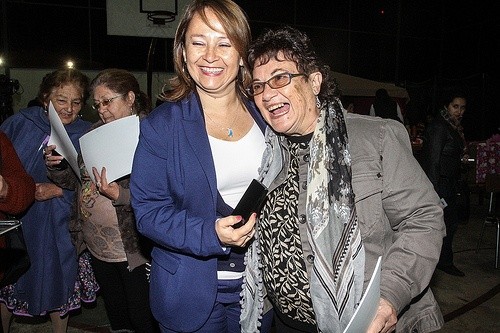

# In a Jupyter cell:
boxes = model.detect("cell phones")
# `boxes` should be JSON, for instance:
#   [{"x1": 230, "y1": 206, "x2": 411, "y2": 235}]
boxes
[{"x1": 229, "y1": 179, "x2": 268, "y2": 229}]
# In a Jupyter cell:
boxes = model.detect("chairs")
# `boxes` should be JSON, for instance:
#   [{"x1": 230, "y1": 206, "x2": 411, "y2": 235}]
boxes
[{"x1": 473, "y1": 174, "x2": 500, "y2": 270}]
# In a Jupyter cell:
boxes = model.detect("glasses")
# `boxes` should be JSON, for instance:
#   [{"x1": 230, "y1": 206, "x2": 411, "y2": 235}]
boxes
[
  {"x1": 246, "y1": 72, "x2": 305, "y2": 97},
  {"x1": 91, "y1": 93, "x2": 124, "y2": 109}
]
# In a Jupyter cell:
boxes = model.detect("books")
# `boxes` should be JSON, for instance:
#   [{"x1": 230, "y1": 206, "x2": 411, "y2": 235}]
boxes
[
  {"x1": 343, "y1": 255, "x2": 383, "y2": 333},
  {"x1": 46, "y1": 100, "x2": 139, "y2": 189}
]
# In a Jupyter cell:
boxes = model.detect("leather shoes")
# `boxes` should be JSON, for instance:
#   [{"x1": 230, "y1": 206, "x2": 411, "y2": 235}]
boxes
[{"x1": 440, "y1": 264, "x2": 464, "y2": 277}]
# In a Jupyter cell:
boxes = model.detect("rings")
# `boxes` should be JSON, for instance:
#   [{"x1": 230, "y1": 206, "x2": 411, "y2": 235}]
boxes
[
  {"x1": 43, "y1": 149, "x2": 51, "y2": 157},
  {"x1": 96, "y1": 182, "x2": 102, "y2": 188}
]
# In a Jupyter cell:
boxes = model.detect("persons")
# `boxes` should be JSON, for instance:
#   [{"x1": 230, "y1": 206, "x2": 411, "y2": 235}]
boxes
[
  {"x1": 0, "y1": 61, "x2": 176, "y2": 333},
  {"x1": 129, "y1": 0, "x2": 275, "y2": 333},
  {"x1": 238, "y1": 26, "x2": 444, "y2": 333},
  {"x1": 340, "y1": 88, "x2": 472, "y2": 277}
]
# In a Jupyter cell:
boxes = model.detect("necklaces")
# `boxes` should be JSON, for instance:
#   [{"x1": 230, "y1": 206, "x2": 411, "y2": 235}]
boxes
[{"x1": 202, "y1": 102, "x2": 245, "y2": 138}]
